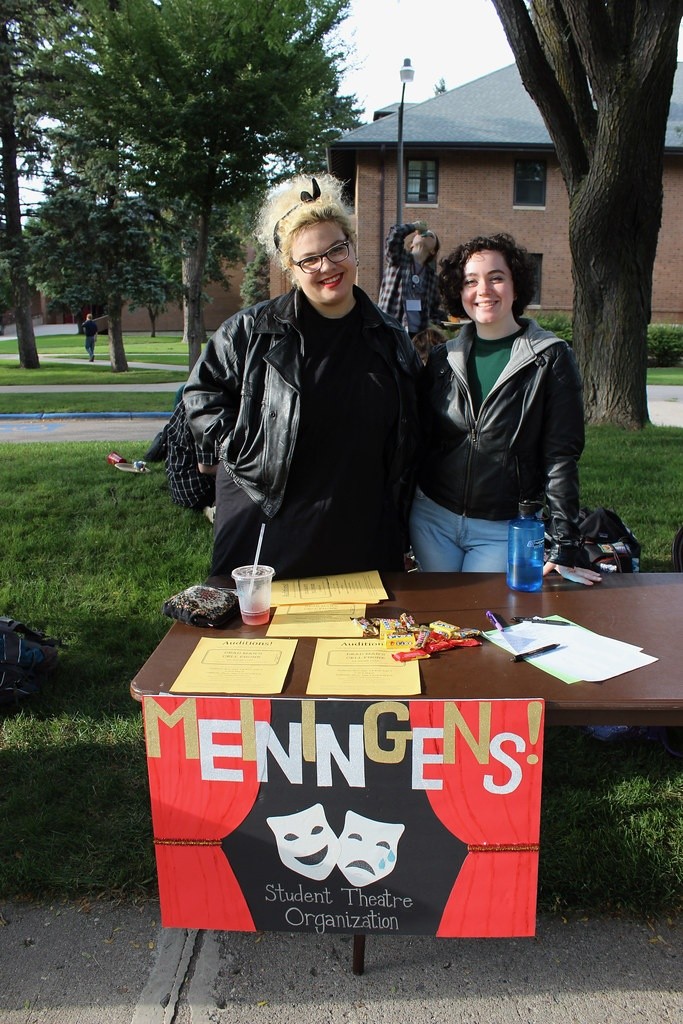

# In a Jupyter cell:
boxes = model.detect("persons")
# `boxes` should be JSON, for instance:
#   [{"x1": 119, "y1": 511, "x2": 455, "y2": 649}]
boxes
[
  {"x1": 182, "y1": 173, "x2": 426, "y2": 578},
  {"x1": 82, "y1": 314, "x2": 98, "y2": 362},
  {"x1": 409, "y1": 233, "x2": 602, "y2": 586},
  {"x1": 165, "y1": 221, "x2": 448, "y2": 511}
]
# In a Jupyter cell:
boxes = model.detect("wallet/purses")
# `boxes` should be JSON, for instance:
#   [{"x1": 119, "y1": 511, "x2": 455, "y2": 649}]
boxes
[{"x1": 165, "y1": 585, "x2": 237, "y2": 628}]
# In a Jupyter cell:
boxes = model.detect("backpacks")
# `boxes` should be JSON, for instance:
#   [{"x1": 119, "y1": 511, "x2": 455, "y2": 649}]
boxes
[
  {"x1": 0, "y1": 616, "x2": 58, "y2": 718},
  {"x1": 577, "y1": 507, "x2": 639, "y2": 574}
]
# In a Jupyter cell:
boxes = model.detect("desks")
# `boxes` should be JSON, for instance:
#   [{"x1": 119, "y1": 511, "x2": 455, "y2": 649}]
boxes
[{"x1": 129, "y1": 573, "x2": 683, "y2": 978}]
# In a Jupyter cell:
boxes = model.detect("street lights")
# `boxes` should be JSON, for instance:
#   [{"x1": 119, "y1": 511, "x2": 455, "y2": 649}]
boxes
[{"x1": 395, "y1": 57, "x2": 415, "y2": 232}]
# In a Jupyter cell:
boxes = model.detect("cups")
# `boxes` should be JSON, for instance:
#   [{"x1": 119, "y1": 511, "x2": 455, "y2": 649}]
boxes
[
  {"x1": 106, "y1": 451, "x2": 127, "y2": 464},
  {"x1": 232, "y1": 565, "x2": 276, "y2": 625}
]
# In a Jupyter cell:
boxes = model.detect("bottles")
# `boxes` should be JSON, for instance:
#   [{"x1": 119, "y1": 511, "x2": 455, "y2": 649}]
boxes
[{"x1": 506, "y1": 500, "x2": 552, "y2": 592}]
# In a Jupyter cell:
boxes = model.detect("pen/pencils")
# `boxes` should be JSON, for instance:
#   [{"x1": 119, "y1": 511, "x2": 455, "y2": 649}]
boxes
[
  {"x1": 509, "y1": 644, "x2": 561, "y2": 663},
  {"x1": 485, "y1": 611, "x2": 505, "y2": 633},
  {"x1": 532, "y1": 620, "x2": 570, "y2": 626}
]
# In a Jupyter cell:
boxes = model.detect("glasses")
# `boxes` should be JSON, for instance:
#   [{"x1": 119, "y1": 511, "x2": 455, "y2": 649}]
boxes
[{"x1": 290, "y1": 234, "x2": 350, "y2": 274}]
